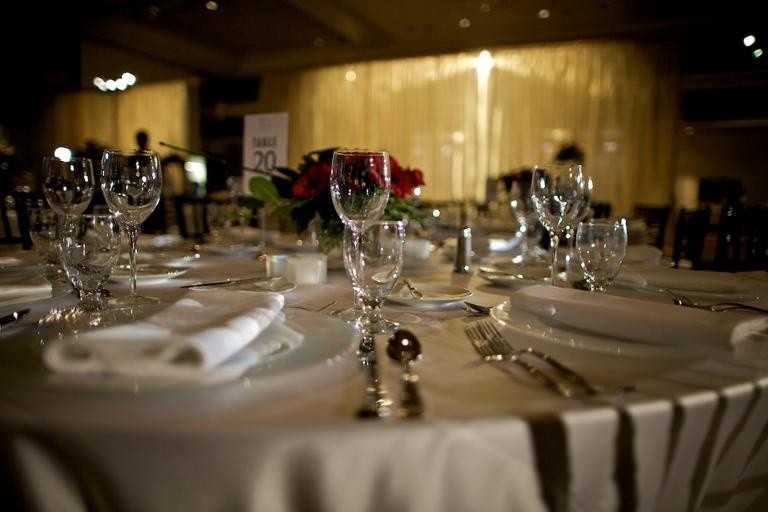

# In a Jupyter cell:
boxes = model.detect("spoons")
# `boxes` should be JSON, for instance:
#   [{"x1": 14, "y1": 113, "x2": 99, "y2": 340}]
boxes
[
  {"x1": 387, "y1": 331, "x2": 422, "y2": 416},
  {"x1": 480, "y1": 266, "x2": 525, "y2": 279},
  {"x1": 403, "y1": 278, "x2": 423, "y2": 299}
]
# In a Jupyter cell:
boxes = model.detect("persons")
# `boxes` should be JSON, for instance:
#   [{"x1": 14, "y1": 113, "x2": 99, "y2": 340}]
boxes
[{"x1": 131, "y1": 126, "x2": 153, "y2": 155}]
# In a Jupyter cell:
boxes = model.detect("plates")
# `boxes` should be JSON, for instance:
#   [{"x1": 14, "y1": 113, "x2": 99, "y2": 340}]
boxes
[
  {"x1": 491, "y1": 299, "x2": 736, "y2": 361},
  {"x1": 384, "y1": 281, "x2": 472, "y2": 304},
  {"x1": 634, "y1": 278, "x2": 751, "y2": 298},
  {"x1": 110, "y1": 228, "x2": 261, "y2": 284},
  {"x1": 481, "y1": 262, "x2": 550, "y2": 284},
  {"x1": 188, "y1": 279, "x2": 297, "y2": 294}
]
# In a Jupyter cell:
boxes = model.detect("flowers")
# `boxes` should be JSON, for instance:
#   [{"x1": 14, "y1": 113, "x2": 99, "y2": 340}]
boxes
[{"x1": 225, "y1": 146, "x2": 436, "y2": 257}]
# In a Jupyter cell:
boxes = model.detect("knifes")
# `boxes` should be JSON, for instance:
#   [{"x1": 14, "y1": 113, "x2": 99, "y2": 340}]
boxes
[{"x1": 355, "y1": 336, "x2": 379, "y2": 419}]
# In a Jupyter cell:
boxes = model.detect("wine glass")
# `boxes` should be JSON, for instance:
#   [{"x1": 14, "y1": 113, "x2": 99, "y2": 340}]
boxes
[
  {"x1": 342, "y1": 219, "x2": 406, "y2": 330},
  {"x1": 330, "y1": 150, "x2": 391, "y2": 318},
  {"x1": 100, "y1": 149, "x2": 163, "y2": 306},
  {"x1": 60, "y1": 216, "x2": 119, "y2": 311},
  {"x1": 508, "y1": 163, "x2": 627, "y2": 291},
  {"x1": 30, "y1": 156, "x2": 94, "y2": 279}
]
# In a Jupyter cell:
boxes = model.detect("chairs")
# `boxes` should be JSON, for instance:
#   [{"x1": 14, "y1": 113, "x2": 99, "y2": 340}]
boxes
[
  {"x1": 539, "y1": 202, "x2": 612, "y2": 247},
  {"x1": 0, "y1": 190, "x2": 50, "y2": 250},
  {"x1": 171, "y1": 194, "x2": 264, "y2": 240},
  {"x1": 674, "y1": 206, "x2": 711, "y2": 269},
  {"x1": 633, "y1": 206, "x2": 672, "y2": 250},
  {"x1": 713, "y1": 203, "x2": 767, "y2": 273}
]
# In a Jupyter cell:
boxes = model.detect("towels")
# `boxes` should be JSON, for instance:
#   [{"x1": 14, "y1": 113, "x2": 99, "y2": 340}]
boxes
[
  {"x1": 38, "y1": 290, "x2": 302, "y2": 387},
  {"x1": 509, "y1": 285, "x2": 767, "y2": 348},
  {"x1": 0, "y1": 272, "x2": 53, "y2": 307},
  {"x1": 614, "y1": 267, "x2": 768, "y2": 301},
  {"x1": 2, "y1": 245, "x2": 22, "y2": 266}
]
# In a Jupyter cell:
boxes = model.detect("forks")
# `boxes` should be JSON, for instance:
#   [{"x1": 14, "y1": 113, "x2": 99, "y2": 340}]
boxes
[
  {"x1": 665, "y1": 289, "x2": 767, "y2": 313},
  {"x1": 285, "y1": 300, "x2": 336, "y2": 312},
  {"x1": 465, "y1": 320, "x2": 594, "y2": 396}
]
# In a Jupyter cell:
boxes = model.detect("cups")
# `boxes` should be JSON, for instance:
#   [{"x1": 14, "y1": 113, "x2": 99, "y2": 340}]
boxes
[
  {"x1": 395, "y1": 238, "x2": 458, "y2": 269},
  {"x1": 264, "y1": 252, "x2": 326, "y2": 284}
]
